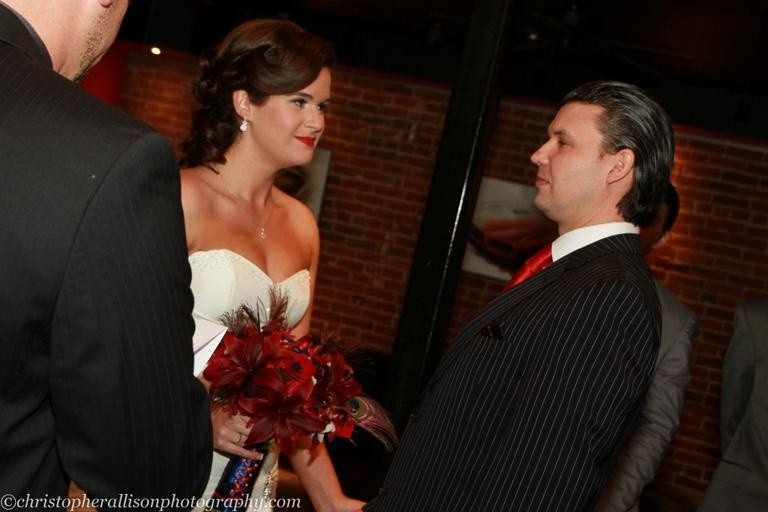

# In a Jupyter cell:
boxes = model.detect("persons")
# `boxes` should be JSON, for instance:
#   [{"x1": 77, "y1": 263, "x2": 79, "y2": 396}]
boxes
[
  {"x1": 0, "y1": 1, "x2": 212, "y2": 512},
  {"x1": 179, "y1": 19, "x2": 368, "y2": 512},
  {"x1": 697, "y1": 301, "x2": 768, "y2": 512}
]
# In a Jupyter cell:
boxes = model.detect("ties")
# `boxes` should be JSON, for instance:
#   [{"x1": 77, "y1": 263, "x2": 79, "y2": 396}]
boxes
[{"x1": 501, "y1": 239, "x2": 552, "y2": 296}]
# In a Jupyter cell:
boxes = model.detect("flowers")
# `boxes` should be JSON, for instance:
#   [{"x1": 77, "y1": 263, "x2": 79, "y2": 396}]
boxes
[{"x1": 209, "y1": 293, "x2": 402, "y2": 467}]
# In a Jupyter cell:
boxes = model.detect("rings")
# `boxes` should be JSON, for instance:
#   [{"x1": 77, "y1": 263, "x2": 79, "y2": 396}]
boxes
[{"x1": 234, "y1": 432, "x2": 242, "y2": 444}]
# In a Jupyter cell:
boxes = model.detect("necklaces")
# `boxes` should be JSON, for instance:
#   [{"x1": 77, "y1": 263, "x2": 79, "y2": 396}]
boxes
[{"x1": 258, "y1": 211, "x2": 274, "y2": 240}]
